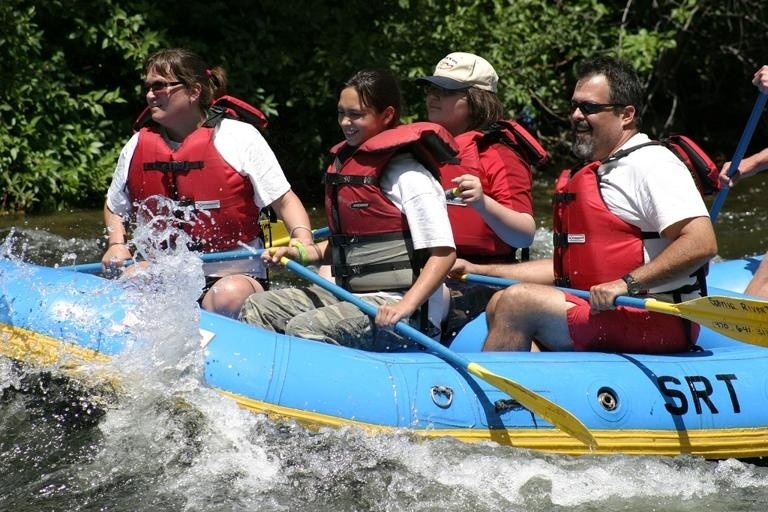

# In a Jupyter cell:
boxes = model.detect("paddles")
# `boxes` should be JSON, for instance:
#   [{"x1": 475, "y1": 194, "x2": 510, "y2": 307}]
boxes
[
  {"x1": 259, "y1": 220, "x2": 330, "y2": 279},
  {"x1": 268, "y1": 250, "x2": 597, "y2": 447},
  {"x1": 452, "y1": 271, "x2": 767, "y2": 346}
]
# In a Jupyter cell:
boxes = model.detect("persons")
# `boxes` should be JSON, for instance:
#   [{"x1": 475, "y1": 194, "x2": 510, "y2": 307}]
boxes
[
  {"x1": 237, "y1": 68, "x2": 458, "y2": 350},
  {"x1": 101, "y1": 48, "x2": 313, "y2": 322},
  {"x1": 719, "y1": 64, "x2": 768, "y2": 301},
  {"x1": 415, "y1": 53, "x2": 537, "y2": 348},
  {"x1": 441, "y1": 59, "x2": 718, "y2": 353}
]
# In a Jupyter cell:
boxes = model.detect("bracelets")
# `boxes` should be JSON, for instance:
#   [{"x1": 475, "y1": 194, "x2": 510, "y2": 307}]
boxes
[
  {"x1": 291, "y1": 241, "x2": 310, "y2": 267},
  {"x1": 309, "y1": 241, "x2": 323, "y2": 264},
  {"x1": 108, "y1": 240, "x2": 127, "y2": 249},
  {"x1": 289, "y1": 224, "x2": 315, "y2": 242}
]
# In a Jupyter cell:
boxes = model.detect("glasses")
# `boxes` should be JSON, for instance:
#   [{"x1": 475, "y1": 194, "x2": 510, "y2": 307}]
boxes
[
  {"x1": 569, "y1": 99, "x2": 626, "y2": 114},
  {"x1": 143, "y1": 80, "x2": 182, "y2": 93}
]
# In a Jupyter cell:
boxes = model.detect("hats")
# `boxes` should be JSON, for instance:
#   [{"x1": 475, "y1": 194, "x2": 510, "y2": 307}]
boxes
[{"x1": 413, "y1": 53, "x2": 498, "y2": 93}]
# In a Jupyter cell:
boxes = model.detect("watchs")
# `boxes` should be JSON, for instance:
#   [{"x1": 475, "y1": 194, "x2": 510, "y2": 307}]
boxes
[{"x1": 621, "y1": 274, "x2": 643, "y2": 298}]
